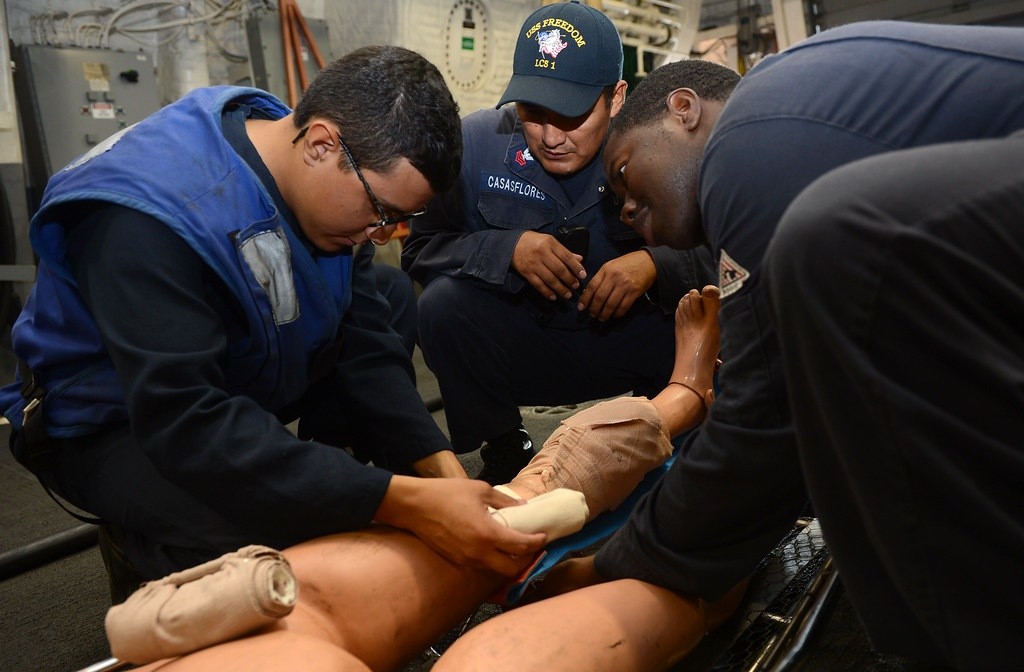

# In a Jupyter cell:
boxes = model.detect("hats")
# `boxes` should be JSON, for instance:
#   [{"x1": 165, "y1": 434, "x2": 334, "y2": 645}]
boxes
[{"x1": 495, "y1": 1, "x2": 624, "y2": 117}]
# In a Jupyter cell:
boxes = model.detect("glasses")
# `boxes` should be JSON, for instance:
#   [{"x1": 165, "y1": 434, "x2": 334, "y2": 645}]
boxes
[{"x1": 291, "y1": 122, "x2": 429, "y2": 226}]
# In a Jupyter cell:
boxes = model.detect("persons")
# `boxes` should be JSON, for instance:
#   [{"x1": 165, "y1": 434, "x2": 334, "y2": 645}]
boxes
[
  {"x1": 16, "y1": 2, "x2": 1024, "y2": 671},
  {"x1": 401, "y1": 0, "x2": 719, "y2": 485}
]
